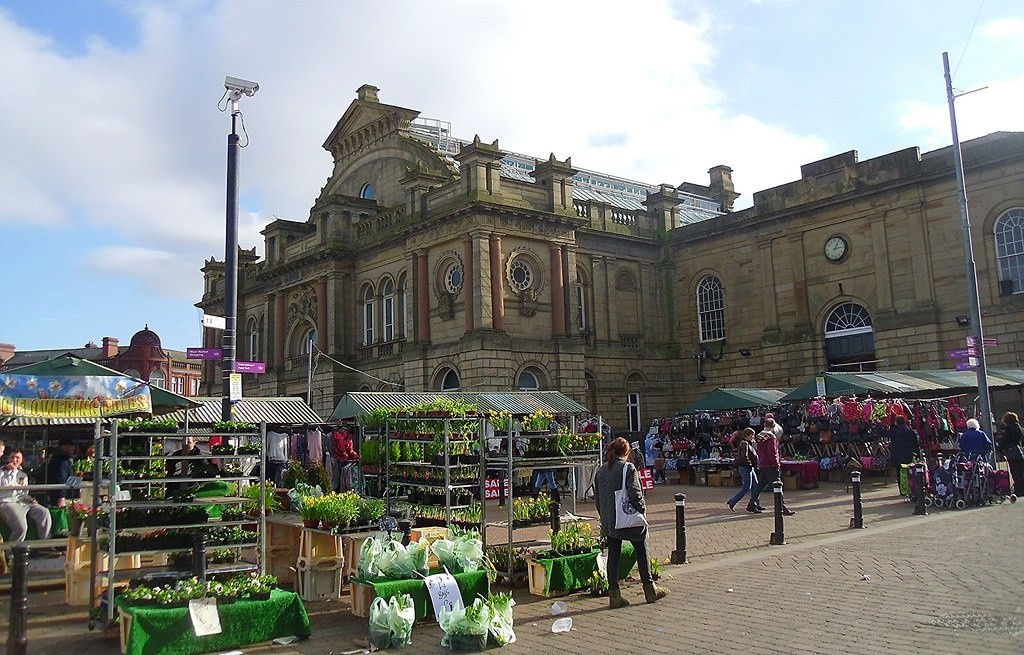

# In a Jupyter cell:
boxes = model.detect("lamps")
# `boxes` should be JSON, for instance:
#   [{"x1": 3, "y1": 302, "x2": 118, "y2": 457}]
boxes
[
  {"x1": 739, "y1": 348, "x2": 751, "y2": 357},
  {"x1": 955, "y1": 315, "x2": 968, "y2": 326}
]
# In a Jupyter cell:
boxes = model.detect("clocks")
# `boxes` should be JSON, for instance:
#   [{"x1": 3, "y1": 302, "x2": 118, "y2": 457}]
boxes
[{"x1": 823, "y1": 233, "x2": 853, "y2": 265}]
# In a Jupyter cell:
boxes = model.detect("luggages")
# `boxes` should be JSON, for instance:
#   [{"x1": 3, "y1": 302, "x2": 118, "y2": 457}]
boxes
[{"x1": 896, "y1": 451, "x2": 1018, "y2": 508}]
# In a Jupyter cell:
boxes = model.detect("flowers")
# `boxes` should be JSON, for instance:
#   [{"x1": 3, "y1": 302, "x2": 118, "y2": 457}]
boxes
[
  {"x1": 488, "y1": 408, "x2": 605, "y2": 459},
  {"x1": 648, "y1": 557, "x2": 677, "y2": 582},
  {"x1": 586, "y1": 570, "x2": 610, "y2": 598},
  {"x1": 65, "y1": 501, "x2": 101, "y2": 519},
  {"x1": 295, "y1": 489, "x2": 360, "y2": 522},
  {"x1": 121, "y1": 572, "x2": 277, "y2": 605},
  {"x1": 498, "y1": 491, "x2": 551, "y2": 520},
  {"x1": 544, "y1": 521, "x2": 603, "y2": 557}
]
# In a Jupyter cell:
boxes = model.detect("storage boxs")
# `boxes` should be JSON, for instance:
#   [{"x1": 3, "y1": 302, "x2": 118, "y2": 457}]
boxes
[
  {"x1": 782, "y1": 475, "x2": 801, "y2": 490},
  {"x1": 694, "y1": 469, "x2": 741, "y2": 487}
]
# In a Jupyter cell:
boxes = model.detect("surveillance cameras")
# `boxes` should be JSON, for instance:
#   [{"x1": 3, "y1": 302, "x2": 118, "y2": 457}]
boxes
[{"x1": 224, "y1": 75, "x2": 260, "y2": 96}]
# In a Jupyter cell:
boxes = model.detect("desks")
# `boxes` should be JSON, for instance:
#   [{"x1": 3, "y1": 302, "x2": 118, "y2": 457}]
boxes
[{"x1": 689, "y1": 461, "x2": 819, "y2": 489}]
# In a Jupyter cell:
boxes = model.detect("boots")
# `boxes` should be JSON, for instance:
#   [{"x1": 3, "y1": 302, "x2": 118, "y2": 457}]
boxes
[
  {"x1": 532, "y1": 487, "x2": 541, "y2": 500},
  {"x1": 552, "y1": 487, "x2": 560, "y2": 502},
  {"x1": 608, "y1": 589, "x2": 631, "y2": 608},
  {"x1": 643, "y1": 582, "x2": 670, "y2": 603}
]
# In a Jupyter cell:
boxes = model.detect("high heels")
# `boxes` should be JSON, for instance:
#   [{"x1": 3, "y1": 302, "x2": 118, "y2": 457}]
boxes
[
  {"x1": 726, "y1": 499, "x2": 736, "y2": 513},
  {"x1": 753, "y1": 505, "x2": 764, "y2": 510}
]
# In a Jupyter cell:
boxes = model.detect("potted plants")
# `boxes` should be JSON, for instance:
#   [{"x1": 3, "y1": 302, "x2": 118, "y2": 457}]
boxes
[
  {"x1": 358, "y1": 396, "x2": 481, "y2": 532},
  {"x1": 441, "y1": 590, "x2": 517, "y2": 652},
  {"x1": 189, "y1": 420, "x2": 259, "y2": 477},
  {"x1": 368, "y1": 590, "x2": 416, "y2": 650},
  {"x1": 94, "y1": 506, "x2": 260, "y2": 569},
  {"x1": 74, "y1": 418, "x2": 179, "y2": 502}
]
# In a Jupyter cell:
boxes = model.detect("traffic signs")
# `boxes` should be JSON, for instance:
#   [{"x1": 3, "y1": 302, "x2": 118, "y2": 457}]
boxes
[
  {"x1": 233, "y1": 360, "x2": 268, "y2": 375},
  {"x1": 954, "y1": 363, "x2": 976, "y2": 371},
  {"x1": 947, "y1": 337, "x2": 980, "y2": 366},
  {"x1": 983, "y1": 338, "x2": 997, "y2": 347},
  {"x1": 203, "y1": 314, "x2": 227, "y2": 330},
  {"x1": 185, "y1": 347, "x2": 223, "y2": 360}
]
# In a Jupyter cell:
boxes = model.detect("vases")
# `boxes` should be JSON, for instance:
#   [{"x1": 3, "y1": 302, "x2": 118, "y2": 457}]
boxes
[
  {"x1": 513, "y1": 516, "x2": 551, "y2": 527},
  {"x1": 518, "y1": 430, "x2": 550, "y2": 435},
  {"x1": 523, "y1": 450, "x2": 599, "y2": 459},
  {"x1": 651, "y1": 570, "x2": 659, "y2": 581},
  {"x1": 494, "y1": 430, "x2": 516, "y2": 437},
  {"x1": 69, "y1": 517, "x2": 84, "y2": 537},
  {"x1": 302, "y1": 519, "x2": 321, "y2": 528},
  {"x1": 590, "y1": 589, "x2": 609, "y2": 598},
  {"x1": 123, "y1": 593, "x2": 271, "y2": 610},
  {"x1": 525, "y1": 547, "x2": 600, "y2": 560},
  {"x1": 321, "y1": 520, "x2": 336, "y2": 530}
]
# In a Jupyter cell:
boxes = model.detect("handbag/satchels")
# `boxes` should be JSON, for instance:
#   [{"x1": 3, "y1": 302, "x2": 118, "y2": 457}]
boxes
[
  {"x1": 615, "y1": 464, "x2": 649, "y2": 529},
  {"x1": 368, "y1": 592, "x2": 414, "y2": 649},
  {"x1": 438, "y1": 596, "x2": 518, "y2": 650},
  {"x1": 358, "y1": 536, "x2": 482, "y2": 577},
  {"x1": 647, "y1": 398, "x2": 968, "y2": 473}
]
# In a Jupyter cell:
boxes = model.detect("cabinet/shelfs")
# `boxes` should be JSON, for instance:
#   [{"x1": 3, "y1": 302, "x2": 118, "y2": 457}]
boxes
[
  {"x1": 88, "y1": 419, "x2": 266, "y2": 637},
  {"x1": 483, "y1": 414, "x2": 603, "y2": 590},
  {"x1": 386, "y1": 418, "x2": 484, "y2": 545}
]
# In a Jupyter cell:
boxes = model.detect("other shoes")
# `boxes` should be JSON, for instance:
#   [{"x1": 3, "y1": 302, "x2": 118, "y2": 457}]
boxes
[
  {"x1": 747, "y1": 506, "x2": 761, "y2": 514},
  {"x1": 782, "y1": 508, "x2": 796, "y2": 516},
  {"x1": 39, "y1": 547, "x2": 63, "y2": 555}
]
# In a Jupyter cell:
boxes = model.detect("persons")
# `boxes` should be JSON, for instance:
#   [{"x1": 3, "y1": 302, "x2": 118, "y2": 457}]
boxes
[
  {"x1": 958, "y1": 418, "x2": 992, "y2": 460},
  {"x1": 726, "y1": 427, "x2": 765, "y2": 511},
  {"x1": 0, "y1": 440, "x2": 62, "y2": 557},
  {"x1": 630, "y1": 433, "x2": 667, "y2": 484},
  {"x1": 166, "y1": 436, "x2": 207, "y2": 500},
  {"x1": 531, "y1": 472, "x2": 560, "y2": 501},
  {"x1": 48, "y1": 439, "x2": 77, "y2": 553},
  {"x1": 746, "y1": 419, "x2": 796, "y2": 515},
  {"x1": 728, "y1": 429, "x2": 745, "y2": 449},
  {"x1": 874, "y1": 415, "x2": 920, "y2": 495},
  {"x1": 763, "y1": 412, "x2": 784, "y2": 492},
  {"x1": 594, "y1": 437, "x2": 669, "y2": 610},
  {"x1": 996, "y1": 412, "x2": 1024, "y2": 497}
]
